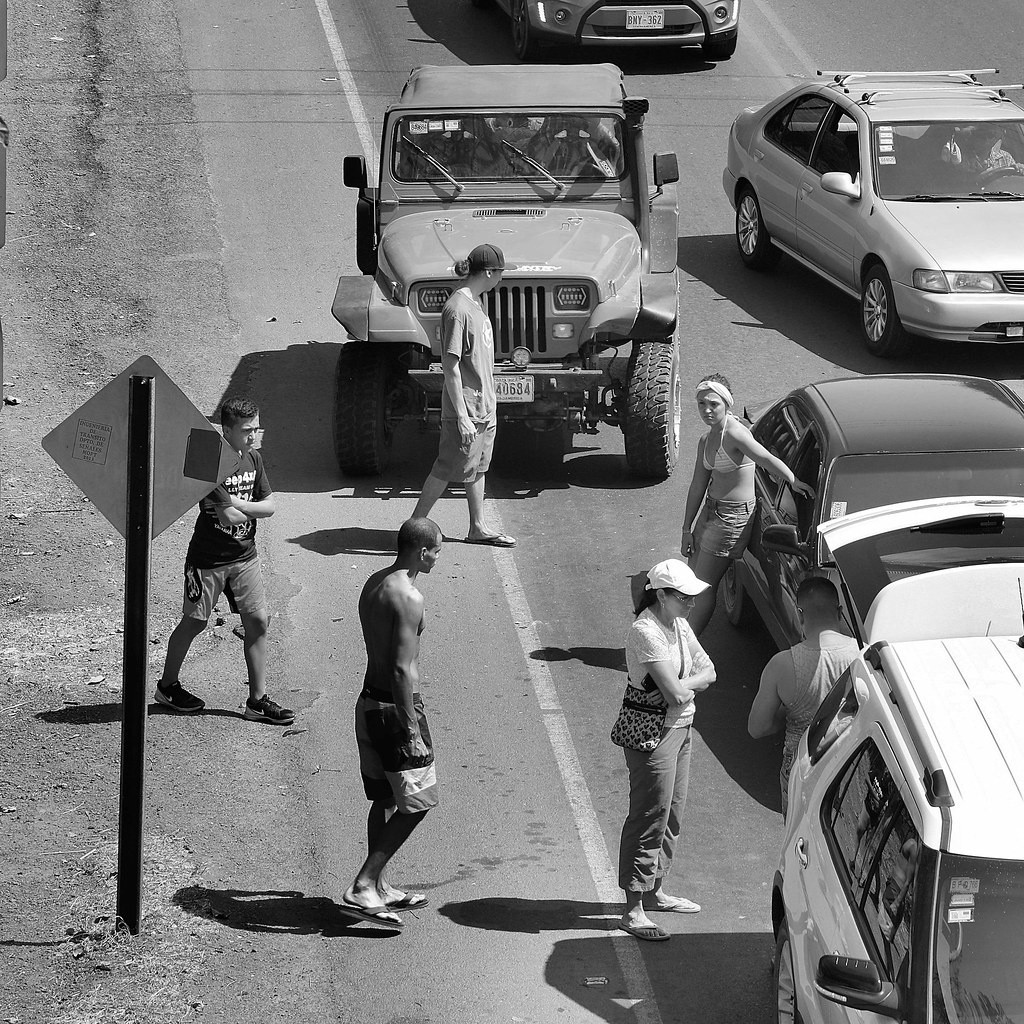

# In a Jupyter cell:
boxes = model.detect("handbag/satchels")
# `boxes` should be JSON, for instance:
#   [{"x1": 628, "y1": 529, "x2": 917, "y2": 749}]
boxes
[{"x1": 611, "y1": 684, "x2": 669, "y2": 752}]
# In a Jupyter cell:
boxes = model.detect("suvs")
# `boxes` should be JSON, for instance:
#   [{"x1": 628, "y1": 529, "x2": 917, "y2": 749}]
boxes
[{"x1": 331, "y1": 63, "x2": 682, "y2": 482}]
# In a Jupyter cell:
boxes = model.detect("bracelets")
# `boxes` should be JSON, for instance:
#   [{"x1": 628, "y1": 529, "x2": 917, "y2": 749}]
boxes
[{"x1": 682, "y1": 529, "x2": 690, "y2": 534}]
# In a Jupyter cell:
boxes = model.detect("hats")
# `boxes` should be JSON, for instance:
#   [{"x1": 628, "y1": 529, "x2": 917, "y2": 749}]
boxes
[
  {"x1": 468, "y1": 244, "x2": 517, "y2": 270},
  {"x1": 644, "y1": 559, "x2": 713, "y2": 596}
]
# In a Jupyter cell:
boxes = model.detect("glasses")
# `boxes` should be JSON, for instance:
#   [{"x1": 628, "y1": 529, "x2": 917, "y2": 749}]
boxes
[{"x1": 664, "y1": 588, "x2": 697, "y2": 604}]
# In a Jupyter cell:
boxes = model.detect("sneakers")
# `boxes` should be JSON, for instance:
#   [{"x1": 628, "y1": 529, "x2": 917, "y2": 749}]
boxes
[
  {"x1": 155, "y1": 679, "x2": 206, "y2": 712},
  {"x1": 244, "y1": 694, "x2": 296, "y2": 724}
]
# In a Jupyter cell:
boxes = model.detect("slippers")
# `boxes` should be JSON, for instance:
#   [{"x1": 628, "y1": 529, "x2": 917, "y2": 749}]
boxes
[
  {"x1": 464, "y1": 532, "x2": 517, "y2": 548},
  {"x1": 618, "y1": 921, "x2": 671, "y2": 941},
  {"x1": 385, "y1": 891, "x2": 430, "y2": 913},
  {"x1": 644, "y1": 897, "x2": 701, "y2": 913},
  {"x1": 339, "y1": 906, "x2": 406, "y2": 929}
]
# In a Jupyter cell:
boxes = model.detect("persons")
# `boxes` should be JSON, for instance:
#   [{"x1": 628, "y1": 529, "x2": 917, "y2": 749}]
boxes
[
  {"x1": 528, "y1": 116, "x2": 620, "y2": 173},
  {"x1": 878, "y1": 830, "x2": 918, "y2": 979},
  {"x1": 849, "y1": 766, "x2": 893, "y2": 873},
  {"x1": 412, "y1": 244, "x2": 516, "y2": 547},
  {"x1": 337, "y1": 518, "x2": 443, "y2": 929},
  {"x1": 948, "y1": 125, "x2": 1024, "y2": 186},
  {"x1": 154, "y1": 396, "x2": 296, "y2": 726},
  {"x1": 618, "y1": 558, "x2": 717, "y2": 940},
  {"x1": 748, "y1": 578, "x2": 869, "y2": 824},
  {"x1": 681, "y1": 374, "x2": 816, "y2": 638}
]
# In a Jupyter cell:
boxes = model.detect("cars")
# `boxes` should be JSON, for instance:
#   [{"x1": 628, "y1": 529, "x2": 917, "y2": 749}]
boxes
[
  {"x1": 497, "y1": 0, "x2": 740, "y2": 63},
  {"x1": 721, "y1": 372, "x2": 1024, "y2": 653},
  {"x1": 723, "y1": 69, "x2": 1024, "y2": 358},
  {"x1": 770, "y1": 492, "x2": 1024, "y2": 1024}
]
